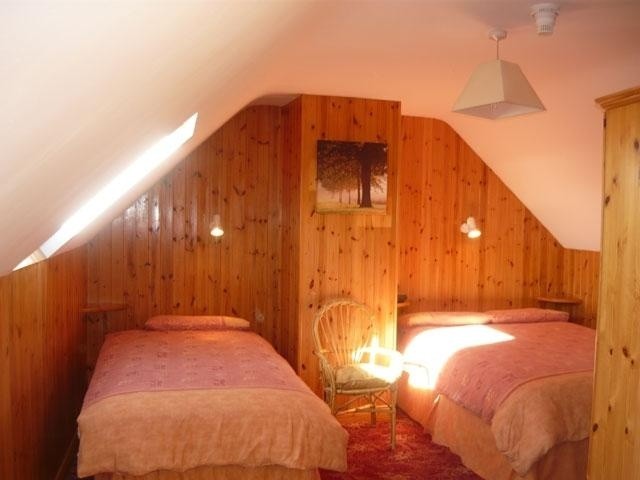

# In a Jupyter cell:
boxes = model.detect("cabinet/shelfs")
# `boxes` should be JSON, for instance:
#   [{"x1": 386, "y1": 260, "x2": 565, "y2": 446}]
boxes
[{"x1": 592, "y1": 85, "x2": 640, "y2": 451}]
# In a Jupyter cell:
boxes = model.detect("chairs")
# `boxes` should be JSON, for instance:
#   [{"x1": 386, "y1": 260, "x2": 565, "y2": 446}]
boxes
[{"x1": 313, "y1": 295, "x2": 401, "y2": 449}]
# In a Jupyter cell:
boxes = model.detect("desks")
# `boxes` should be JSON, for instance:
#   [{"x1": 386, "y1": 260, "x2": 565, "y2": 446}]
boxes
[{"x1": 538, "y1": 296, "x2": 582, "y2": 321}]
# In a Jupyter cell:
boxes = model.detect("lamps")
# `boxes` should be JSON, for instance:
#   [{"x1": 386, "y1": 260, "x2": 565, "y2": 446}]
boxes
[
  {"x1": 208, "y1": 215, "x2": 224, "y2": 237},
  {"x1": 460, "y1": 217, "x2": 482, "y2": 240},
  {"x1": 450, "y1": 29, "x2": 546, "y2": 120}
]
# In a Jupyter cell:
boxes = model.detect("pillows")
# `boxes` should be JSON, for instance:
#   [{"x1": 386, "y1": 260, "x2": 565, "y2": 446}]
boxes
[
  {"x1": 410, "y1": 313, "x2": 492, "y2": 326},
  {"x1": 489, "y1": 308, "x2": 571, "y2": 324},
  {"x1": 145, "y1": 314, "x2": 250, "y2": 330}
]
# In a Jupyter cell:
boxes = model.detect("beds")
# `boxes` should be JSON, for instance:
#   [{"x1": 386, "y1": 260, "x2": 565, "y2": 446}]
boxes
[
  {"x1": 76, "y1": 327, "x2": 347, "y2": 479},
  {"x1": 395, "y1": 318, "x2": 598, "y2": 478}
]
